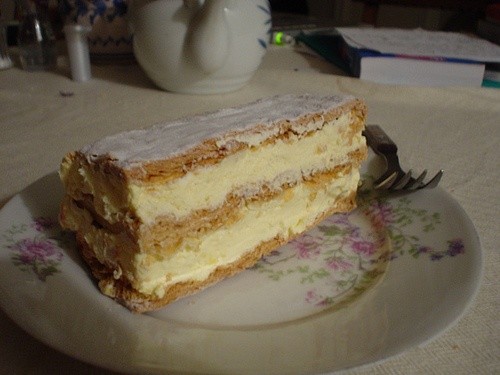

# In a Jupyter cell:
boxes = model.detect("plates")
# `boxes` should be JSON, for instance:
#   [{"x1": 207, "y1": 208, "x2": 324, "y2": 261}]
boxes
[{"x1": 1, "y1": 146, "x2": 485, "y2": 371}]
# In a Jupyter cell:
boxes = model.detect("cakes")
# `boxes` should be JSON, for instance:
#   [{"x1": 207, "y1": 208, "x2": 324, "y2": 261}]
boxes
[{"x1": 58, "y1": 92, "x2": 367, "y2": 313}]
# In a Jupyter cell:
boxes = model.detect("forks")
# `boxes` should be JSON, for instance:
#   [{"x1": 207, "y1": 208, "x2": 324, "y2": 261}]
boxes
[{"x1": 364, "y1": 121, "x2": 445, "y2": 194}]
